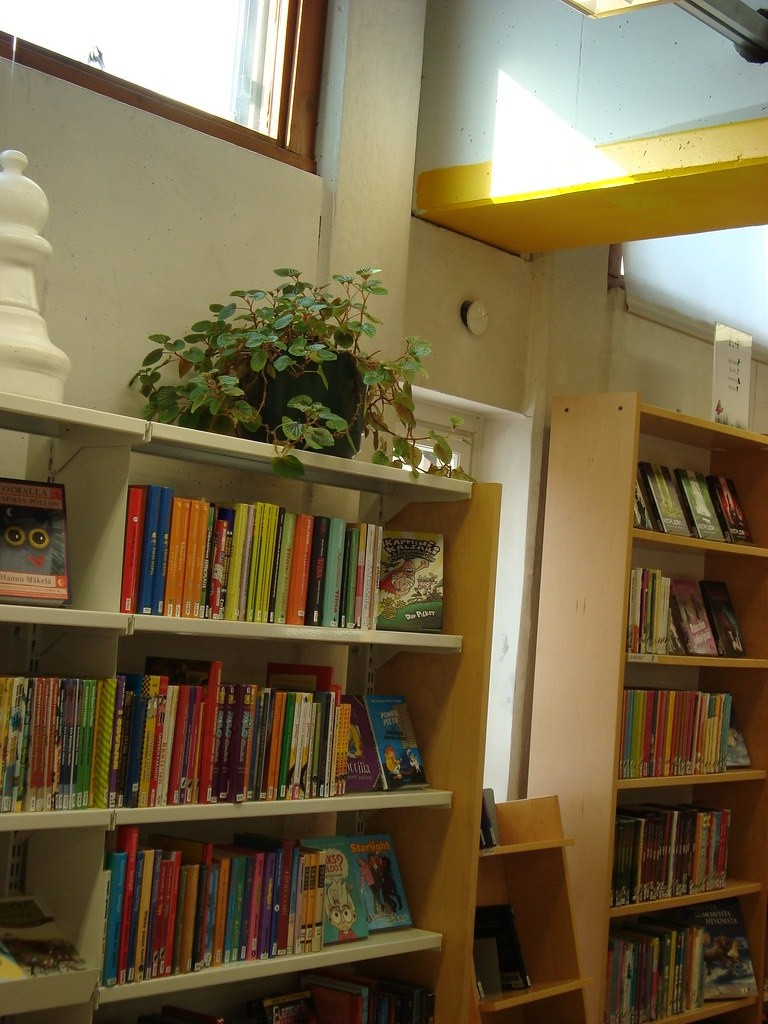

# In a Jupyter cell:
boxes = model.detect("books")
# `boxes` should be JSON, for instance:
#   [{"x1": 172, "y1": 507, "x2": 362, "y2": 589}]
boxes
[
  {"x1": 602, "y1": 897, "x2": 758, "y2": 1024},
  {"x1": 120, "y1": 484, "x2": 445, "y2": 632},
  {"x1": 0, "y1": 893, "x2": 89, "y2": 978},
  {"x1": 616, "y1": 685, "x2": 752, "y2": 779},
  {"x1": 133, "y1": 959, "x2": 437, "y2": 1024},
  {"x1": 466, "y1": 902, "x2": 533, "y2": 1001},
  {"x1": 101, "y1": 825, "x2": 411, "y2": 988},
  {"x1": 1, "y1": 473, "x2": 70, "y2": 608},
  {"x1": 479, "y1": 789, "x2": 503, "y2": 850},
  {"x1": 609, "y1": 799, "x2": 735, "y2": 908},
  {"x1": 632, "y1": 461, "x2": 756, "y2": 548},
  {"x1": 623, "y1": 566, "x2": 747, "y2": 658},
  {"x1": 0, "y1": 658, "x2": 434, "y2": 812}
]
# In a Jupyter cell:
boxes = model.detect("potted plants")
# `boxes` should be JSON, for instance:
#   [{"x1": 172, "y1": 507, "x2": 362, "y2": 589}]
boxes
[{"x1": 129, "y1": 267, "x2": 478, "y2": 481}]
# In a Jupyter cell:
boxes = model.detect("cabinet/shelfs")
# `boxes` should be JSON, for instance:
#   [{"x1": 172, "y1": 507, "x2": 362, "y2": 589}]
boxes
[
  {"x1": 470, "y1": 794, "x2": 594, "y2": 1024},
  {"x1": 529, "y1": 393, "x2": 768, "y2": 1024},
  {"x1": 1, "y1": 394, "x2": 503, "y2": 1024}
]
